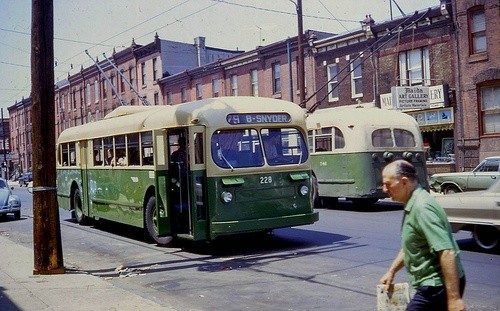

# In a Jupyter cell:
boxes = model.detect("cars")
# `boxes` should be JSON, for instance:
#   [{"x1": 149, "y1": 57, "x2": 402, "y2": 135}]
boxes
[
  {"x1": 431, "y1": 157, "x2": 500, "y2": 195},
  {"x1": 0, "y1": 178, "x2": 21, "y2": 220}
]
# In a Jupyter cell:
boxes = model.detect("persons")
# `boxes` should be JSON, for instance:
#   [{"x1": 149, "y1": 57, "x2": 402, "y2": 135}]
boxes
[
  {"x1": 213, "y1": 131, "x2": 242, "y2": 164},
  {"x1": 261, "y1": 133, "x2": 288, "y2": 162},
  {"x1": 83, "y1": 135, "x2": 189, "y2": 166},
  {"x1": 381, "y1": 160, "x2": 466, "y2": 311}
]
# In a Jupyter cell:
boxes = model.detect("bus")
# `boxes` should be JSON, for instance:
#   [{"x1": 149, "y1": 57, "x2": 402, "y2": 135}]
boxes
[
  {"x1": 56, "y1": 95, "x2": 320, "y2": 247},
  {"x1": 306, "y1": 98, "x2": 430, "y2": 208}
]
